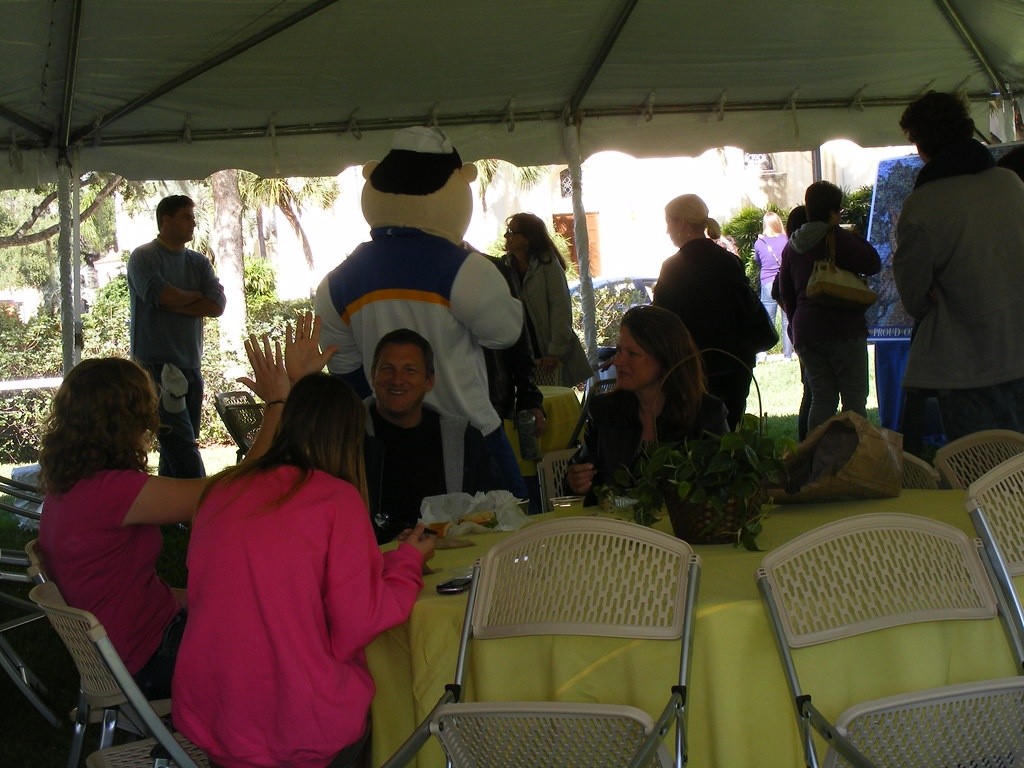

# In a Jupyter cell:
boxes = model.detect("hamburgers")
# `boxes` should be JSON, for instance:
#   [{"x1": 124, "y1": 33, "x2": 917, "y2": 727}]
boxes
[{"x1": 460, "y1": 512, "x2": 498, "y2": 529}]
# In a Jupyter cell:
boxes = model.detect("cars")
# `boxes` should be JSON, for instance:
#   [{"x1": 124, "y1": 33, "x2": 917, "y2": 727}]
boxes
[{"x1": 566, "y1": 276, "x2": 657, "y2": 343}]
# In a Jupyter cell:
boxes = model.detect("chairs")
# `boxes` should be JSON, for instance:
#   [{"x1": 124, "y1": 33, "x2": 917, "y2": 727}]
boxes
[{"x1": 0, "y1": 391, "x2": 1024, "y2": 768}]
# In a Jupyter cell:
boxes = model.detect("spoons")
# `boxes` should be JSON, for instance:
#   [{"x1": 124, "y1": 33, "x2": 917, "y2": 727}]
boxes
[{"x1": 374, "y1": 513, "x2": 438, "y2": 535}]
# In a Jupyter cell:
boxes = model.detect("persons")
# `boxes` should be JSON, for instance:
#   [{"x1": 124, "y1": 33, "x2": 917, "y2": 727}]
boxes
[
  {"x1": 561, "y1": 305, "x2": 727, "y2": 507},
  {"x1": 893, "y1": 89, "x2": 1024, "y2": 461},
  {"x1": 40, "y1": 333, "x2": 290, "y2": 700},
  {"x1": 756, "y1": 181, "x2": 882, "y2": 443},
  {"x1": 283, "y1": 311, "x2": 500, "y2": 546},
  {"x1": 651, "y1": 194, "x2": 778, "y2": 433},
  {"x1": 130, "y1": 194, "x2": 227, "y2": 478},
  {"x1": 172, "y1": 372, "x2": 437, "y2": 768},
  {"x1": 458, "y1": 212, "x2": 594, "y2": 437}
]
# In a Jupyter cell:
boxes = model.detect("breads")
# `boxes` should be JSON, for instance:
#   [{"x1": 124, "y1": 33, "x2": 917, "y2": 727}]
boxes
[{"x1": 425, "y1": 520, "x2": 456, "y2": 538}]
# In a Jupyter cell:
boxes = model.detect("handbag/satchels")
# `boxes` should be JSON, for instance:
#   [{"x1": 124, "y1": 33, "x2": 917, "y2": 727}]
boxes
[
  {"x1": 726, "y1": 250, "x2": 779, "y2": 354},
  {"x1": 806, "y1": 226, "x2": 877, "y2": 305}
]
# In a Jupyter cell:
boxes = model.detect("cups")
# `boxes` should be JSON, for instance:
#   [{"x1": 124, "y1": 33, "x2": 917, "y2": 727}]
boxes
[
  {"x1": 549, "y1": 496, "x2": 584, "y2": 517},
  {"x1": 518, "y1": 499, "x2": 530, "y2": 516}
]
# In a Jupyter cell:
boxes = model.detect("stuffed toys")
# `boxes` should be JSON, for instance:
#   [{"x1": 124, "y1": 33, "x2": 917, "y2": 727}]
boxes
[{"x1": 313, "y1": 126, "x2": 526, "y2": 497}]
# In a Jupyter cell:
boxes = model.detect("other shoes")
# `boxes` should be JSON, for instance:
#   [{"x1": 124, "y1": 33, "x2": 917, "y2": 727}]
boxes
[{"x1": 758, "y1": 356, "x2": 766, "y2": 364}]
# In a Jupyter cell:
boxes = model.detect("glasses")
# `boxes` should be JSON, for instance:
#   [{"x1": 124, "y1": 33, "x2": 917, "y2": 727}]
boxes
[{"x1": 506, "y1": 229, "x2": 523, "y2": 237}]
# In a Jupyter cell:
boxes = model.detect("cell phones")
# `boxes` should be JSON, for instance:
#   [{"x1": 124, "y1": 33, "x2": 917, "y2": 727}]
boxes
[{"x1": 436, "y1": 577, "x2": 472, "y2": 594}]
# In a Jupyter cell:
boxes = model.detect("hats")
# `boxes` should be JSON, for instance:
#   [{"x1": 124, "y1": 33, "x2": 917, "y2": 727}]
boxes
[{"x1": 160, "y1": 364, "x2": 188, "y2": 413}]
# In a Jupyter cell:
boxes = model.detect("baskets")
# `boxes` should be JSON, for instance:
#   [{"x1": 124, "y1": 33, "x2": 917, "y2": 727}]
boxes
[{"x1": 653, "y1": 349, "x2": 762, "y2": 545}]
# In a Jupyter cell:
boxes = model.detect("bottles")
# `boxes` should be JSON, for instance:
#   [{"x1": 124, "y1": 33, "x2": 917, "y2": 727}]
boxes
[{"x1": 517, "y1": 409, "x2": 541, "y2": 459}]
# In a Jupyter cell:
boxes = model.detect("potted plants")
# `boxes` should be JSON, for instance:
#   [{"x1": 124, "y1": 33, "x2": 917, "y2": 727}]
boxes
[{"x1": 619, "y1": 412, "x2": 799, "y2": 553}]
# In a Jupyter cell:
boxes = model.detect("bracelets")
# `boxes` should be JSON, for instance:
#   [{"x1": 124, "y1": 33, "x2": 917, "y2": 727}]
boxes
[{"x1": 268, "y1": 399, "x2": 286, "y2": 408}]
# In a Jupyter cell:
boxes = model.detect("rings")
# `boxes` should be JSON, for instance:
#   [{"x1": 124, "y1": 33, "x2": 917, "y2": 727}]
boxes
[{"x1": 543, "y1": 418, "x2": 546, "y2": 421}]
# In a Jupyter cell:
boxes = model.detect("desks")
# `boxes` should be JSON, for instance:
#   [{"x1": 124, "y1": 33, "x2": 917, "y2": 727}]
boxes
[
  {"x1": 503, "y1": 383, "x2": 589, "y2": 478},
  {"x1": 358, "y1": 486, "x2": 1024, "y2": 768}
]
